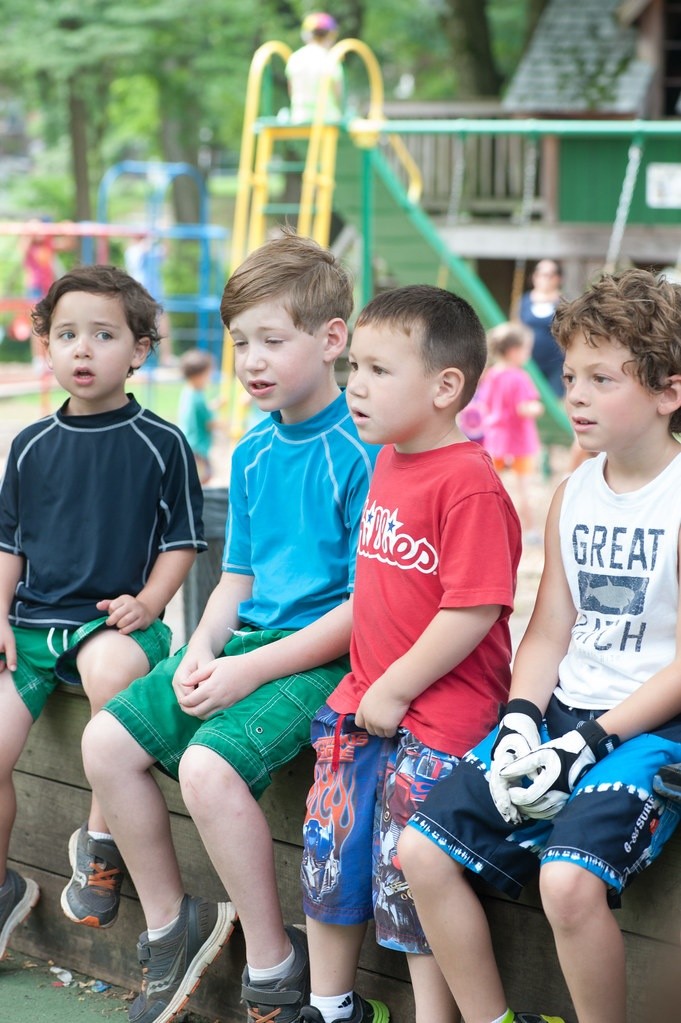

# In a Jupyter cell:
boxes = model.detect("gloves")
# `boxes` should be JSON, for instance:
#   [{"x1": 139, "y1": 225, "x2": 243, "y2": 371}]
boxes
[
  {"x1": 488, "y1": 698, "x2": 542, "y2": 827},
  {"x1": 499, "y1": 720, "x2": 620, "y2": 820}
]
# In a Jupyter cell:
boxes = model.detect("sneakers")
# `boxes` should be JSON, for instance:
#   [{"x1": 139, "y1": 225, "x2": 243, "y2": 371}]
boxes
[
  {"x1": 0, "y1": 867, "x2": 40, "y2": 960},
  {"x1": 128, "y1": 894, "x2": 238, "y2": 1023},
  {"x1": 300, "y1": 991, "x2": 390, "y2": 1023},
  {"x1": 60, "y1": 822, "x2": 128, "y2": 929},
  {"x1": 503, "y1": 1008, "x2": 565, "y2": 1023},
  {"x1": 241, "y1": 924, "x2": 311, "y2": 1023}
]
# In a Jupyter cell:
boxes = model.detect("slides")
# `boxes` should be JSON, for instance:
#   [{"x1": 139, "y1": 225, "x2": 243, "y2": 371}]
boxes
[{"x1": 262, "y1": 111, "x2": 576, "y2": 481}]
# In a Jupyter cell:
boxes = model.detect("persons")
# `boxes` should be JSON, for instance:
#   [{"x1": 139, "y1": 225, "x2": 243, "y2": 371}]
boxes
[
  {"x1": 0, "y1": 216, "x2": 681, "y2": 1022},
  {"x1": 279, "y1": 9, "x2": 347, "y2": 120}
]
